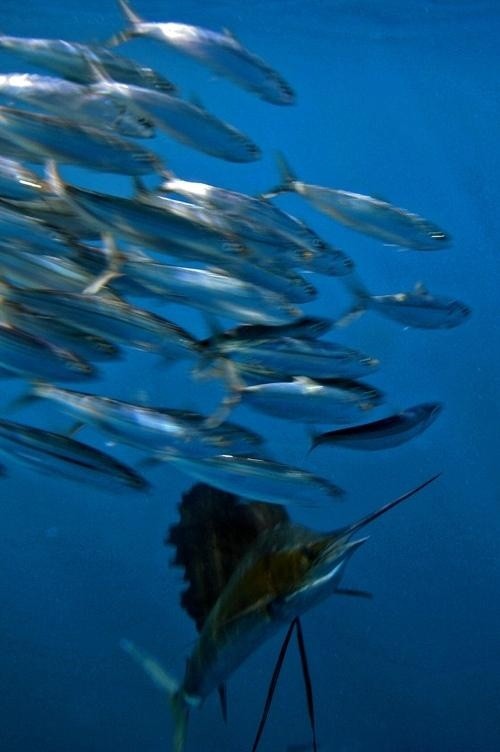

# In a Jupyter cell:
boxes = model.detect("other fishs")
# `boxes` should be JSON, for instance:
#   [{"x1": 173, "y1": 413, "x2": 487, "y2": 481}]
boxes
[{"x1": 0, "y1": 0, "x2": 472, "y2": 752}]
